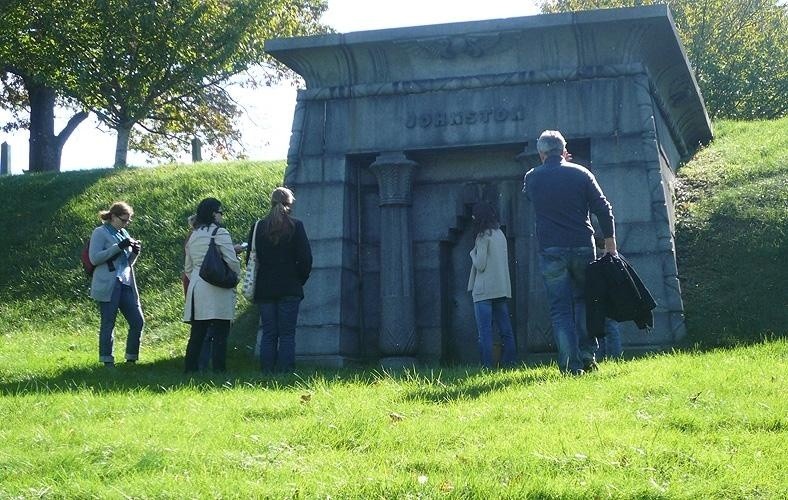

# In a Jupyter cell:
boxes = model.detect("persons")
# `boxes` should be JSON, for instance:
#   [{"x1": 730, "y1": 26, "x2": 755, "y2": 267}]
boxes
[
  {"x1": 522, "y1": 129, "x2": 619, "y2": 378},
  {"x1": 466, "y1": 201, "x2": 518, "y2": 371},
  {"x1": 183, "y1": 213, "x2": 246, "y2": 376},
  {"x1": 87, "y1": 201, "x2": 145, "y2": 369},
  {"x1": 180, "y1": 197, "x2": 243, "y2": 374},
  {"x1": 243, "y1": 188, "x2": 315, "y2": 377}
]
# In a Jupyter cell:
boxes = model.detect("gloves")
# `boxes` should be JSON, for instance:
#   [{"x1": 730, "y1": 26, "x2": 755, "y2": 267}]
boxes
[{"x1": 118, "y1": 238, "x2": 141, "y2": 254}]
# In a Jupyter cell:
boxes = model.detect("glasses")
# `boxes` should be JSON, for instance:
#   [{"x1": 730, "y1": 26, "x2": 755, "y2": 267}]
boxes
[{"x1": 117, "y1": 216, "x2": 128, "y2": 223}]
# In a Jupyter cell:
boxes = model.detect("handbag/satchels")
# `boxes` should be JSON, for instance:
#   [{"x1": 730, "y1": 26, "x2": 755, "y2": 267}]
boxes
[
  {"x1": 199, "y1": 238, "x2": 239, "y2": 288},
  {"x1": 241, "y1": 251, "x2": 260, "y2": 301},
  {"x1": 83, "y1": 239, "x2": 95, "y2": 277}
]
